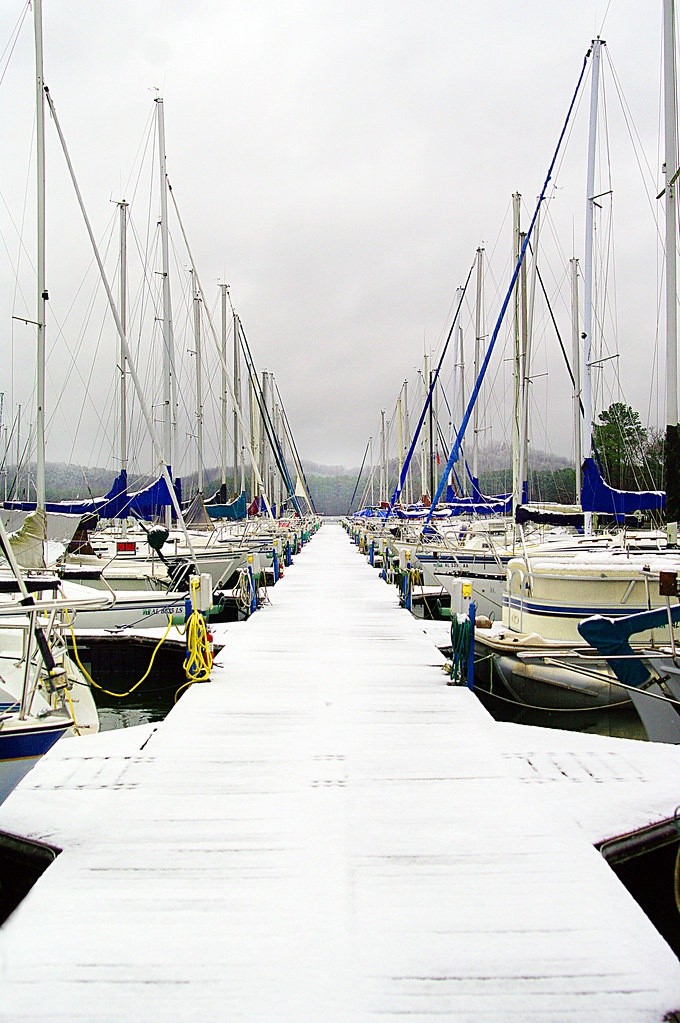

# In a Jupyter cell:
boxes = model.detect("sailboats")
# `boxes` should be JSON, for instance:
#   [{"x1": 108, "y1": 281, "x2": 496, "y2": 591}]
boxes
[
  {"x1": 0, "y1": 0, "x2": 322, "y2": 803},
  {"x1": 341, "y1": 0, "x2": 680, "y2": 747}
]
